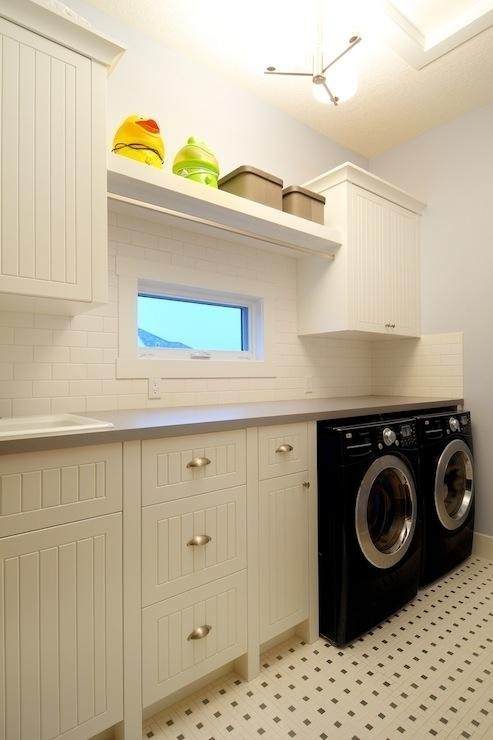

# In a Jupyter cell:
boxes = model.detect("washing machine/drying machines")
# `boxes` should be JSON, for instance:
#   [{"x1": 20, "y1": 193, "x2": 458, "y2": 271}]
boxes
[
  {"x1": 416, "y1": 409, "x2": 474, "y2": 590},
  {"x1": 317, "y1": 415, "x2": 419, "y2": 645}
]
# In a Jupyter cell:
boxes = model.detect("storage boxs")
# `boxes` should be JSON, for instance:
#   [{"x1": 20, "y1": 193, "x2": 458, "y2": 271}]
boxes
[{"x1": 217, "y1": 164, "x2": 326, "y2": 226}]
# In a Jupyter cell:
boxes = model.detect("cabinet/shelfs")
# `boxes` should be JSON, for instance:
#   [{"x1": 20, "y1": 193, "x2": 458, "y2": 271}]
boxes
[
  {"x1": 298, "y1": 160, "x2": 426, "y2": 341},
  {"x1": 0, "y1": 443, "x2": 124, "y2": 740},
  {"x1": 0, "y1": 15, "x2": 110, "y2": 318},
  {"x1": 251, "y1": 414, "x2": 320, "y2": 680},
  {"x1": 123, "y1": 428, "x2": 251, "y2": 739}
]
